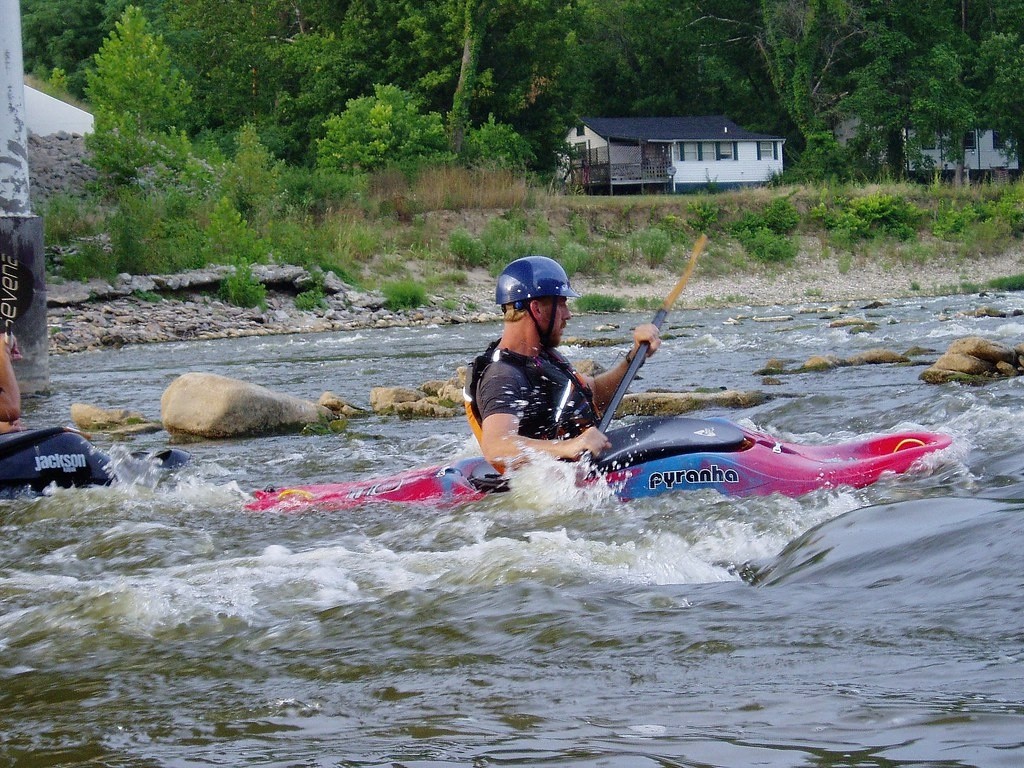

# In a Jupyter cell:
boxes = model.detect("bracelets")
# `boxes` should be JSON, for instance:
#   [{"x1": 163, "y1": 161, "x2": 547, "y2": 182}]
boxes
[{"x1": 627, "y1": 351, "x2": 645, "y2": 369}]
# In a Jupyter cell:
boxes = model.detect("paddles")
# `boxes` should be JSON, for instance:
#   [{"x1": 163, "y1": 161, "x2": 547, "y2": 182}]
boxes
[{"x1": 577, "y1": 230, "x2": 709, "y2": 483}]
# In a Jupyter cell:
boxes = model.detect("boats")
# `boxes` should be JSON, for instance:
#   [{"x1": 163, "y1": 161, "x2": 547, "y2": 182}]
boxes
[
  {"x1": 0, "y1": 425, "x2": 196, "y2": 500},
  {"x1": 243, "y1": 415, "x2": 953, "y2": 516}
]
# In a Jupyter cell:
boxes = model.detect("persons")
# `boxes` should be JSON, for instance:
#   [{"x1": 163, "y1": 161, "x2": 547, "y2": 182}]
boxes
[
  {"x1": 0, "y1": 319, "x2": 26, "y2": 434},
  {"x1": 462, "y1": 256, "x2": 660, "y2": 471}
]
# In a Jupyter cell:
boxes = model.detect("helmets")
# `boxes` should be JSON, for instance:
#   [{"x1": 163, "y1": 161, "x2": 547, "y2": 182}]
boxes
[{"x1": 495, "y1": 256, "x2": 582, "y2": 304}]
